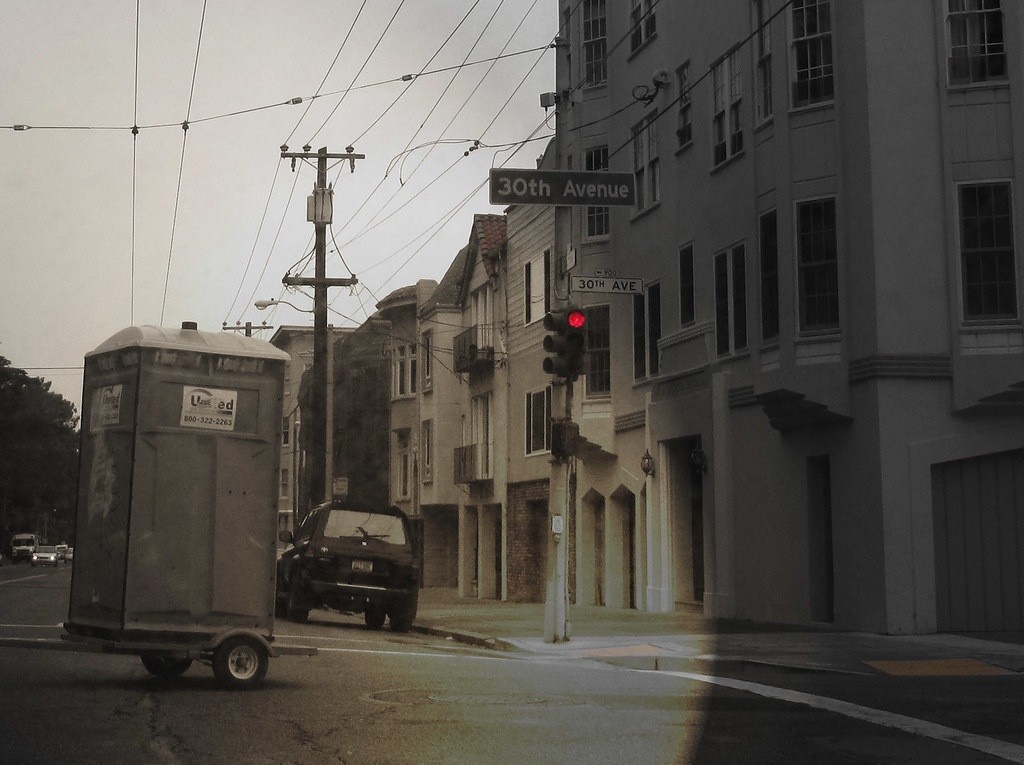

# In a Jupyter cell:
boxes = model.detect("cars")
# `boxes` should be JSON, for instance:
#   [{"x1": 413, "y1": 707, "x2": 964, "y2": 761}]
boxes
[
  {"x1": 32, "y1": 546, "x2": 60, "y2": 567},
  {"x1": 55, "y1": 545, "x2": 74, "y2": 563}
]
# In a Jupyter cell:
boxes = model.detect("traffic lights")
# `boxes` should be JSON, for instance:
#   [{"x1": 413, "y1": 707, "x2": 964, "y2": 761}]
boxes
[
  {"x1": 563, "y1": 307, "x2": 586, "y2": 371},
  {"x1": 542, "y1": 309, "x2": 565, "y2": 377}
]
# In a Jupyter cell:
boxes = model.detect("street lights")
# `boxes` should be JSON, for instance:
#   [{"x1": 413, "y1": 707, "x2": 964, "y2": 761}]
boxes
[{"x1": 254, "y1": 297, "x2": 327, "y2": 508}]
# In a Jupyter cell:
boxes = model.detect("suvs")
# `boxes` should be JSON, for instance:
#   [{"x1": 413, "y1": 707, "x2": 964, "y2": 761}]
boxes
[
  {"x1": 276, "y1": 500, "x2": 420, "y2": 633},
  {"x1": 10, "y1": 533, "x2": 39, "y2": 558}
]
